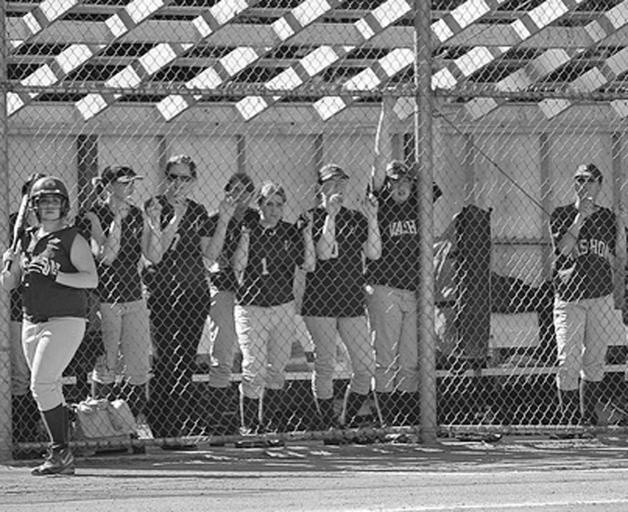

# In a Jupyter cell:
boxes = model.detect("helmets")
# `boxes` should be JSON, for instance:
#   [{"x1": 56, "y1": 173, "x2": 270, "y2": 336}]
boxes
[{"x1": 28, "y1": 176, "x2": 69, "y2": 201}]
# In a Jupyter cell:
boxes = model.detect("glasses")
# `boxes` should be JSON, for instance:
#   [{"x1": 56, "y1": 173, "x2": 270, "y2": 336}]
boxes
[{"x1": 167, "y1": 173, "x2": 192, "y2": 183}]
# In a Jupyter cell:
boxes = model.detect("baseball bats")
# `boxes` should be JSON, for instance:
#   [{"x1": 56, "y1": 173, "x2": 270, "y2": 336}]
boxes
[{"x1": 1, "y1": 171, "x2": 38, "y2": 278}]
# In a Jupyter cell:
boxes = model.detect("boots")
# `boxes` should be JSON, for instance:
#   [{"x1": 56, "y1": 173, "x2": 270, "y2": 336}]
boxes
[
  {"x1": 31, "y1": 401, "x2": 75, "y2": 476},
  {"x1": 237, "y1": 388, "x2": 420, "y2": 449},
  {"x1": 89, "y1": 379, "x2": 238, "y2": 453},
  {"x1": 548, "y1": 380, "x2": 601, "y2": 439}
]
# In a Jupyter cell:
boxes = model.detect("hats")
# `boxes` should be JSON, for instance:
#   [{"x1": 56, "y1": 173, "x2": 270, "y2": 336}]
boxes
[
  {"x1": 385, "y1": 159, "x2": 415, "y2": 180},
  {"x1": 573, "y1": 163, "x2": 603, "y2": 181},
  {"x1": 317, "y1": 163, "x2": 350, "y2": 183},
  {"x1": 101, "y1": 164, "x2": 144, "y2": 186}
]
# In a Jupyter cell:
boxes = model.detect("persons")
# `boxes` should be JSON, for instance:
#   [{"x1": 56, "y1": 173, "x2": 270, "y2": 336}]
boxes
[
  {"x1": 140, "y1": 152, "x2": 239, "y2": 453},
  {"x1": 209, "y1": 167, "x2": 262, "y2": 448},
  {"x1": 0, "y1": 176, "x2": 101, "y2": 478},
  {"x1": 288, "y1": 164, "x2": 385, "y2": 447},
  {"x1": 231, "y1": 178, "x2": 319, "y2": 452},
  {"x1": 546, "y1": 161, "x2": 628, "y2": 437},
  {"x1": 8, "y1": 173, "x2": 63, "y2": 460},
  {"x1": 362, "y1": 152, "x2": 442, "y2": 444},
  {"x1": 76, "y1": 163, "x2": 164, "y2": 457}
]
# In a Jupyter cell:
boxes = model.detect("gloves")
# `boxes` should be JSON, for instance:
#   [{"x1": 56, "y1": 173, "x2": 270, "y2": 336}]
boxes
[{"x1": 22, "y1": 254, "x2": 61, "y2": 282}]
